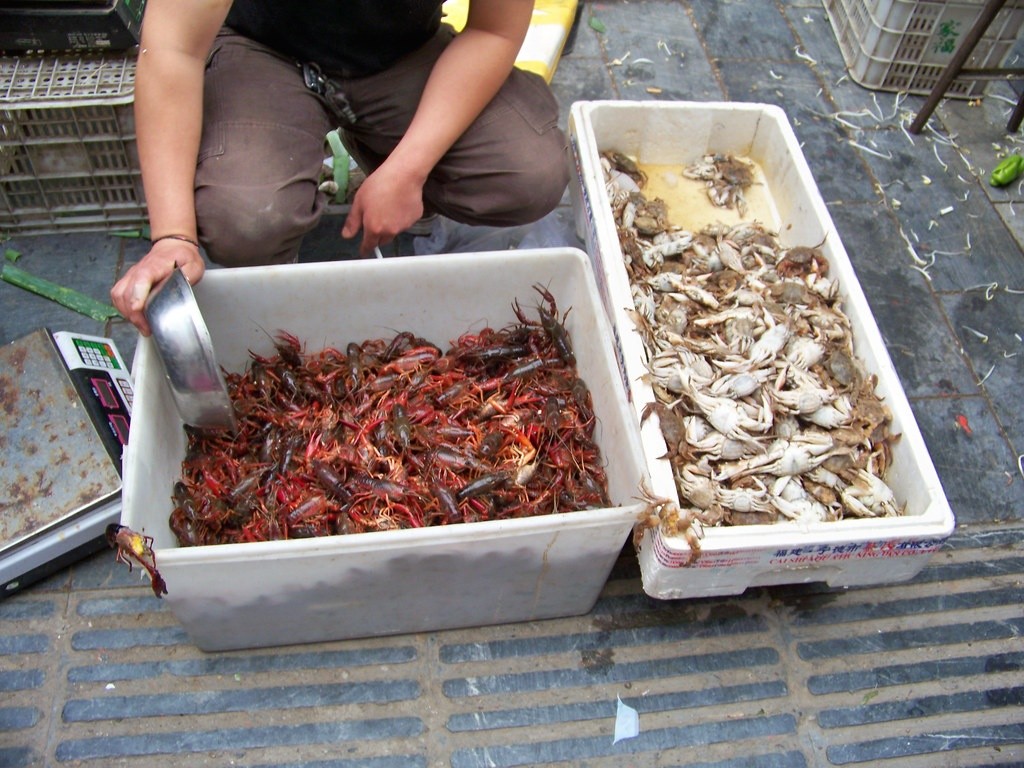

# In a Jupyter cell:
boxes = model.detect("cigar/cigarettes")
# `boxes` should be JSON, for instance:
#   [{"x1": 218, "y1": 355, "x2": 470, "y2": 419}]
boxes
[{"x1": 371, "y1": 245, "x2": 385, "y2": 259}]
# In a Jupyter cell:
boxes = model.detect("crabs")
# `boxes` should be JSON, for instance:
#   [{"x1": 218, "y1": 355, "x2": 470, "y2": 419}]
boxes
[{"x1": 597, "y1": 142, "x2": 909, "y2": 565}]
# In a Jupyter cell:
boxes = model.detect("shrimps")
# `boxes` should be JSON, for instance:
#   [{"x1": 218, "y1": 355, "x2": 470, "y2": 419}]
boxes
[{"x1": 107, "y1": 282, "x2": 618, "y2": 600}]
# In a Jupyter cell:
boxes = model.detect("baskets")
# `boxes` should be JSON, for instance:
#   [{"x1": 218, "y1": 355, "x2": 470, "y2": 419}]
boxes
[
  {"x1": 821, "y1": 0, "x2": 1021, "y2": 100},
  {"x1": 0, "y1": 49, "x2": 153, "y2": 239}
]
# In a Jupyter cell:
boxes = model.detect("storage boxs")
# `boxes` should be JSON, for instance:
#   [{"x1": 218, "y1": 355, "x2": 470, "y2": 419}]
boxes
[
  {"x1": 574, "y1": 99, "x2": 955, "y2": 601},
  {"x1": 0, "y1": 50, "x2": 149, "y2": 236},
  {"x1": 822, "y1": 1, "x2": 1023, "y2": 100},
  {"x1": 119, "y1": 247, "x2": 647, "y2": 652}
]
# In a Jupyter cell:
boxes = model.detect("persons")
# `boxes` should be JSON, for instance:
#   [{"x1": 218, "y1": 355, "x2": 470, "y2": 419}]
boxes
[{"x1": 110, "y1": 0, "x2": 569, "y2": 335}]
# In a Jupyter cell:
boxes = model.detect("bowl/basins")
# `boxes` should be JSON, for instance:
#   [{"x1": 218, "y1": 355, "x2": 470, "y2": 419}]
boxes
[{"x1": 140, "y1": 258, "x2": 240, "y2": 437}]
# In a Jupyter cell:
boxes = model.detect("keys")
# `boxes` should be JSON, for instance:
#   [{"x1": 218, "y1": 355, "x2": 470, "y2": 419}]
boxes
[{"x1": 304, "y1": 61, "x2": 357, "y2": 124}]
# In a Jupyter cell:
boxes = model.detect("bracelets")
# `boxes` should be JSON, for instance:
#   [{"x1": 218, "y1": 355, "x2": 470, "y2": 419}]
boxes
[{"x1": 150, "y1": 236, "x2": 201, "y2": 250}]
